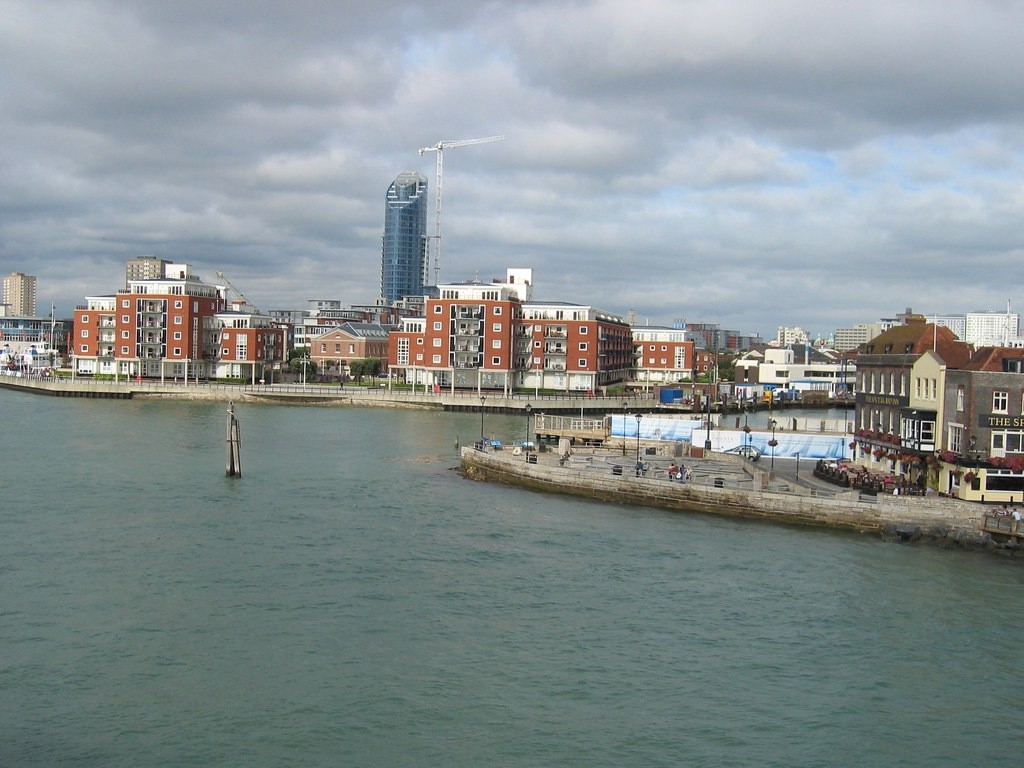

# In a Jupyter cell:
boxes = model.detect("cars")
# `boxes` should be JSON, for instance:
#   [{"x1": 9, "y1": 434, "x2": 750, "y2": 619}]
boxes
[{"x1": 724, "y1": 444, "x2": 761, "y2": 462}]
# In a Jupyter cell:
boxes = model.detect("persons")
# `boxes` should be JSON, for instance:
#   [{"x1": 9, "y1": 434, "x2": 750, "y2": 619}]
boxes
[
  {"x1": 993, "y1": 505, "x2": 1021, "y2": 522},
  {"x1": 39, "y1": 368, "x2": 58, "y2": 381},
  {"x1": 680, "y1": 465, "x2": 696, "y2": 484},
  {"x1": 861, "y1": 465, "x2": 867, "y2": 475},
  {"x1": 635, "y1": 457, "x2": 645, "y2": 478},
  {"x1": 339, "y1": 381, "x2": 345, "y2": 391},
  {"x1": 884, "y1": 476, "x2": 891, "y2": 486},
  {"x1": 668, "y1": 463, "x2": 675, "y2": 483},
  {"x1": 830, "y1": 461, "x2": 837, "y2": 473},
  {"x1": 823, "y1": 461, "x2": 828, "y2": 473}
]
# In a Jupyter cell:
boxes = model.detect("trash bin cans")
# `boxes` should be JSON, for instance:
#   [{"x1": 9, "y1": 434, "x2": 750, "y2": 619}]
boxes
[
  {"x1": 714, "y1": 478, "x2": 725, "y2": 488},
  {"x1": 612, "y1": 465, "x2": 623, "y2": 476},
  {"x1": 529, "y1": 454, "x2": 538, "y2": 464}
]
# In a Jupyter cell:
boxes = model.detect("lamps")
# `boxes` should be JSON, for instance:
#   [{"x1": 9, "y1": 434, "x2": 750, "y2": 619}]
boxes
[{"x1": 969, "y1": 434, "x2": 977, "y2": 447}]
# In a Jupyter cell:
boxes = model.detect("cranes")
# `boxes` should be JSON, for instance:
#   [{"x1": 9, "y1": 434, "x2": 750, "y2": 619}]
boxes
[{"x1": 416, "y1": 133, "x2": 507, "y2": 285}]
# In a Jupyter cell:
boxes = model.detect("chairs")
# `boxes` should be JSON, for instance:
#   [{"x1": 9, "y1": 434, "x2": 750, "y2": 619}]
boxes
[{"x1": 512, "y1": 446, "x2": 522, "y2": 461}]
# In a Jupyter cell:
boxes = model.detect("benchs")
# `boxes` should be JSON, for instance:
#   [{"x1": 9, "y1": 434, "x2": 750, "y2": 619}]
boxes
[
  {"x1": 837, "y1": 463, "x2": 894, "y2": 484},
  {"x1": 564, "y1": 461, "x2": 571, "y2": 467}
]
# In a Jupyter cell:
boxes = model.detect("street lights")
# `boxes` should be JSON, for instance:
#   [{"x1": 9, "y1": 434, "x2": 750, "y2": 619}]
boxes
[
  {"x1": 635, "y1": 413, "x2": 644, "y2": 466},
  {"x1": 525, "y1": 402, "x2": 532, "y2": 463},
  {"x1": 479, "y1": 396, "x2": 486, "y2": 442},
  {"x1": 744, "y1": 407, "x2": 749, "y2": 458},
  {"x1": 623, "y1": 402, "x2": 628, "y2": 457},
  {"x1": 771, "y1": 420, "x2": 778, "y2": 470}
]
